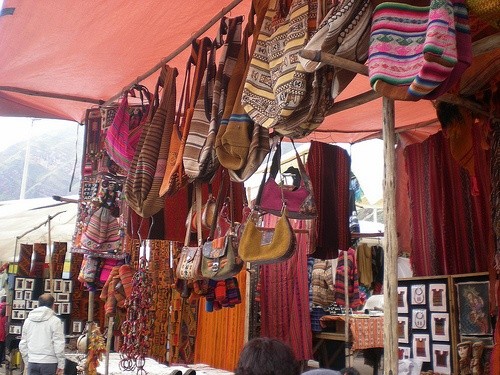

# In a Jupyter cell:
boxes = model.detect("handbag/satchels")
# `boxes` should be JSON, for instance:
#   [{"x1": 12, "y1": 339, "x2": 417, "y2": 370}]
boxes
[{"x1": 68, "y1": 0, "x2": 473, "y2": 282}]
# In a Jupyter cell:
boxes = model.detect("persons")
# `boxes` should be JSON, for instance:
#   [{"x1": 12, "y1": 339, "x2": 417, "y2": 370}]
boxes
[
  {"x1": 19, "y1": 294, "x2": 65, "y2": 375},
  {"x1": 235, "y1": 337, "x2": 301, "y2": 375},
  {"x1": 0, "y1": 296, "x2": 7, "y2": 367}
]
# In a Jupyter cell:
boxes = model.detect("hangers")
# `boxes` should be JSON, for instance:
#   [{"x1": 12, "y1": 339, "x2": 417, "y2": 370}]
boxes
[
  {"x1": 116, "y1": 8, "x2": 245, "y2": 104},
  {"x1": 358, "y1": 236, "x2": 383, "y2": 250}
]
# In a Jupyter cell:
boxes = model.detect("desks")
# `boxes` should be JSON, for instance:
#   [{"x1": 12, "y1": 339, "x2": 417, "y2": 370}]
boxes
[{"x1": 312, "y1": 316, "x2": 385, "y2": 368}]
